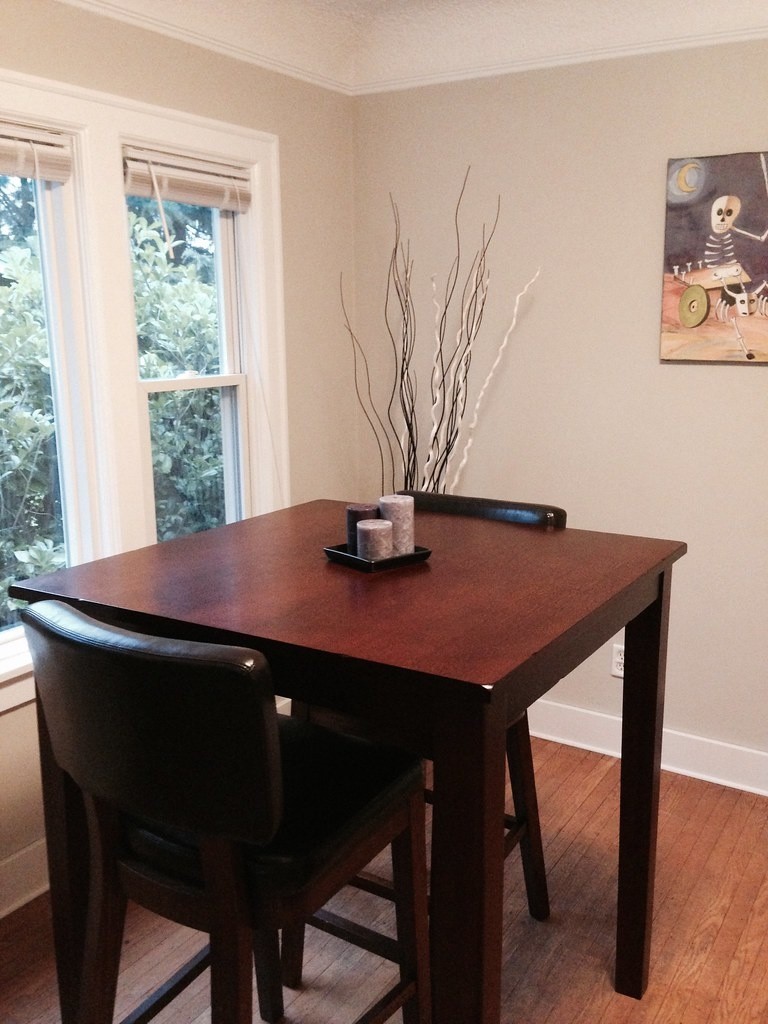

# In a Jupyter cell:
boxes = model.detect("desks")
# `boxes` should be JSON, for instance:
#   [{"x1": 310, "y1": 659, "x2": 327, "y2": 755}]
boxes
[{"x1": 8, "y1": 500, "x2": 688, "y2": 1024}]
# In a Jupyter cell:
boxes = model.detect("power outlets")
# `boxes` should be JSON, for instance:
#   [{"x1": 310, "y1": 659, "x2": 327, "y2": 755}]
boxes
[{"x1": 611, "y1": 643, "x2": 624, "y2": 678}]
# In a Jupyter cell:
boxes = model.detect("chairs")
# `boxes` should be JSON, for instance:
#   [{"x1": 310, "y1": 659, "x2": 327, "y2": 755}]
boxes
[
  {"x1": 17, "y1": 597, "x2": 429, "y2": 1024},
  {"x1": 280, "y1": 490, "x2": 566, "y2": 989}
]
